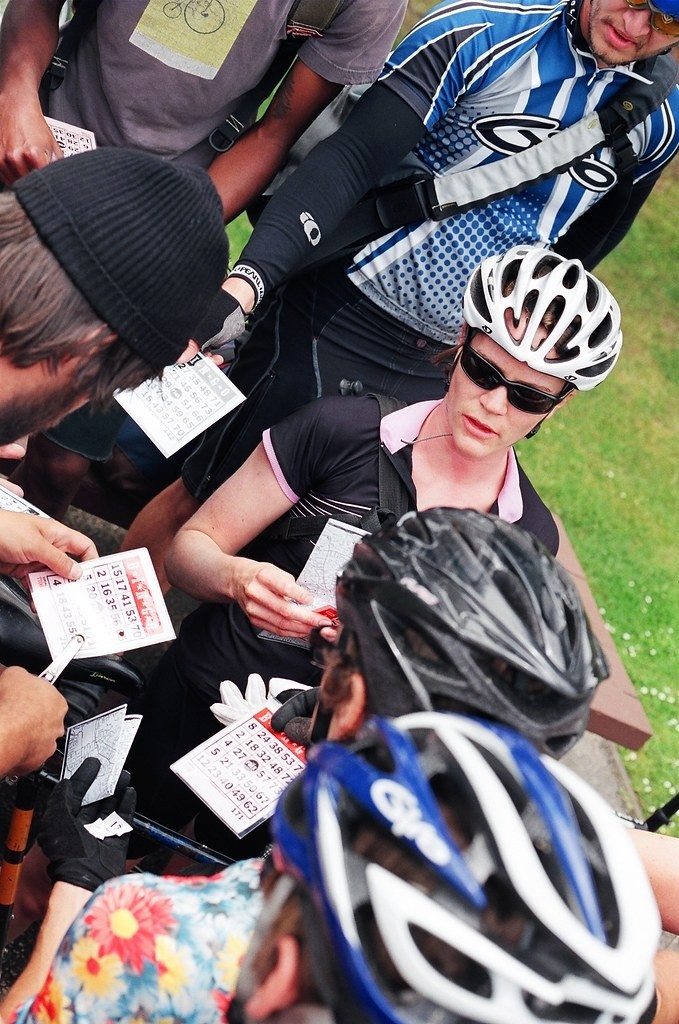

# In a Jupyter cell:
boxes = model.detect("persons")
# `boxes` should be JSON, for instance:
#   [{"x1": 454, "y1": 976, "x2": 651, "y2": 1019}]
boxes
[{"x1": 0, "y1": 0, "x2": 679, "y2": 1024}]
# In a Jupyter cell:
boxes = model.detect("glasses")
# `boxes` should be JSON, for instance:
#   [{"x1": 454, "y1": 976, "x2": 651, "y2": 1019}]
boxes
[
  {"x1": 626, "y1": 0, "x2": 679, "y2": 36},
  {"x1": 460, "y1": 326, "x2": 575, "y2": 416},
  {"x1": 258, "y1": 841, "x2": 312, "y2": 899}
]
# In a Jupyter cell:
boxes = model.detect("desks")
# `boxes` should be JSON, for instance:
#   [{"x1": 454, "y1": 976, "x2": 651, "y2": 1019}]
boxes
[{"x1": 553, "y1": 512, "x2": 651, "y2": 753}]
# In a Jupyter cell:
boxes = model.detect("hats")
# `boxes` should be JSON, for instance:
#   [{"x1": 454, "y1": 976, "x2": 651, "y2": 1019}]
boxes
[
  {"x1": 13, "y1": 149, "x2": 230, "y2": 373},
  {"x1": 651, "y1": 0, "x2": 679, "y2": 19}
]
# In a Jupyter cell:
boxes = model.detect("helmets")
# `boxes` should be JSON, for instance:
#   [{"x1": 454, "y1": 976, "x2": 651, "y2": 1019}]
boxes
[
  {"x1": 272, "y1": 708, "x2": 662, "y2": 1024},
  {"x1": 461, "y1": 244, "x2": 623, "y2": 391},
  {"x1": 337, "y1": 505, "x2": 612, "y2": 762}
]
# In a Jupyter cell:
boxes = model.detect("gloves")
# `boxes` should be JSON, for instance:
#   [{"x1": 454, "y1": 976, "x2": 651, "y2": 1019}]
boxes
[
  {"x1": 210, "y1": 673, "x2": 314, "y2": 726},
  {"x1": 38, "y1": 758, "x2": 138, "y2": 894},
  {"x1": 271, "y1": 686, "x2": 321, "y2": 746}
]
